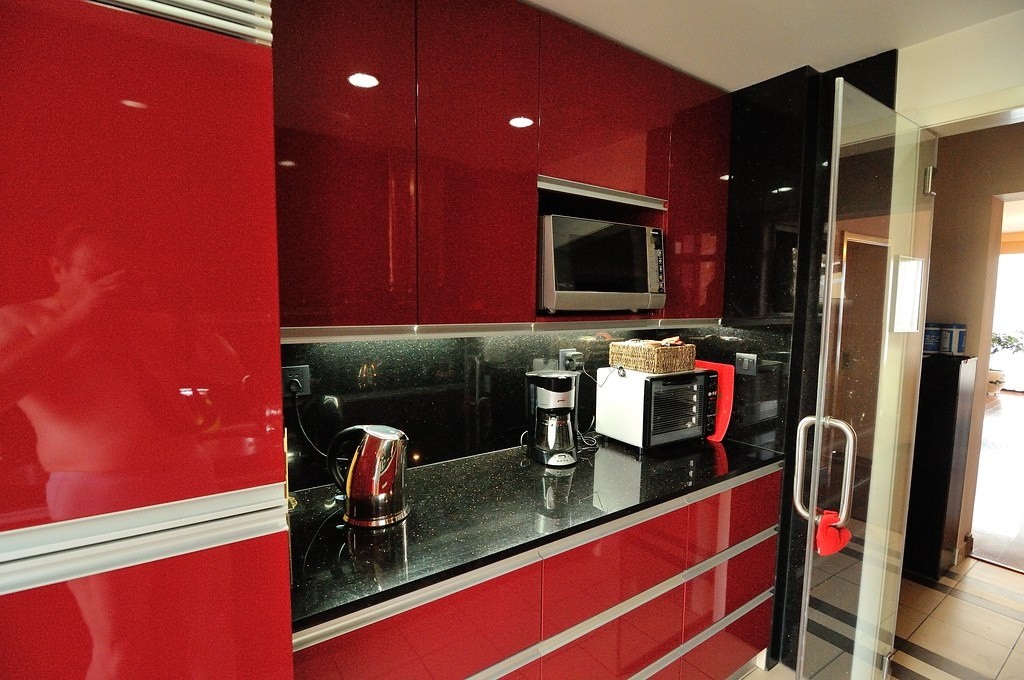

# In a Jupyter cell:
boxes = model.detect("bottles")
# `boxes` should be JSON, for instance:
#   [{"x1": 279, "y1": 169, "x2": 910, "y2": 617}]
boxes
[
  {"x1": 923, "y1": 322, "x2": 943, "y2": 354},
  {"x1": 939, "y1": 324, "x2": 967, "y2": 357}
]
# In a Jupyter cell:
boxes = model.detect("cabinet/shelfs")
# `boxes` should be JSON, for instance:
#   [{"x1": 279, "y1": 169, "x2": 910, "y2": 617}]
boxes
[{"x1": 268, "y1": 1, "x2": 733, "y2": 346}]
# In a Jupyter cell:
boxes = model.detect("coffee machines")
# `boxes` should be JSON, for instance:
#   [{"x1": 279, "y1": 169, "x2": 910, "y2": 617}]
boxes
[{"x1": 524, "y1": 369, "x2": 581, "y2": 470}]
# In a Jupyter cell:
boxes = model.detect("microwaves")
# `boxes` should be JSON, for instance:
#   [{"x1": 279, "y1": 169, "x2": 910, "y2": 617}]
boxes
[{"x1": 537, "y1": 213, "x2": 667, "y2": 314}]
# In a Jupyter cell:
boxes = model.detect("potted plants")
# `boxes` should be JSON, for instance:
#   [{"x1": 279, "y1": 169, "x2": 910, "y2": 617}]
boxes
[{"x1": 988, "y1": 332, "x2": 1023, "y2": 398}]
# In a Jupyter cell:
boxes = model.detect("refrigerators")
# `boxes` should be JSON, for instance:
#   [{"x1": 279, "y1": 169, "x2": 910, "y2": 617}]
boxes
[{"x1": 0, "y1": 0, "x2": 294, "y2": 680}]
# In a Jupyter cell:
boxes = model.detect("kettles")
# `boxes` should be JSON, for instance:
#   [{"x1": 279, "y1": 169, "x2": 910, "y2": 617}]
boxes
[{"x1": 326, "y1": 424, "x2": 410, "y2": 535}]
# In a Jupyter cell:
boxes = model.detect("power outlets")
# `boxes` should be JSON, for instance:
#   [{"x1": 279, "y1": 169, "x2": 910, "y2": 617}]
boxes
[
  {"x1": 282, "y1": 364, "x2": 311, "y2": 398},
  {"x1": 559, "y1": 348, "x2": 582, "y2": 375}
]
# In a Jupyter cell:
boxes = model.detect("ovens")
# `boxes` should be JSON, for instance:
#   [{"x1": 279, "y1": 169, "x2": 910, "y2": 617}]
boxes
[{"x1": 594, "y1": 366, "x2": 720, "y2": 455}]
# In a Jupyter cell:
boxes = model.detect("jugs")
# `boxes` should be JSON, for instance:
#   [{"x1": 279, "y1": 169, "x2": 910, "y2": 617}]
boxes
[{"x1": 533, "y1": 407, "x2": 575, "y2": 453}]
